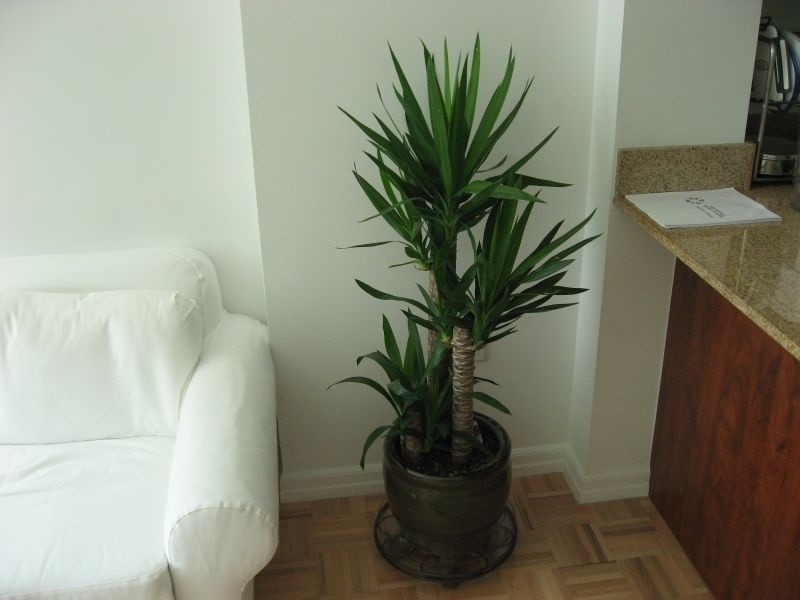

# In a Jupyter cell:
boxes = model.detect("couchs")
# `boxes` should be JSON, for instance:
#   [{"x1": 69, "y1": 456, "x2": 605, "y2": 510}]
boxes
[{"x1": 0, "y1": 246, "x2": 280, "y2": 600}]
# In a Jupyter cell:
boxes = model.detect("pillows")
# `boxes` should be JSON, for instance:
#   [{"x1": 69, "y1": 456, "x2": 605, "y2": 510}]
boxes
[{"x1": 0, "y1": 289, "x2": 203, "y2": 446}]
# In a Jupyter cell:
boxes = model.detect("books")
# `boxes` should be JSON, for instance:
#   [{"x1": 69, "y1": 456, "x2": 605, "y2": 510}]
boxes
[{"x1": 624, "y1": 187, "x2": 782, "y2": 229}]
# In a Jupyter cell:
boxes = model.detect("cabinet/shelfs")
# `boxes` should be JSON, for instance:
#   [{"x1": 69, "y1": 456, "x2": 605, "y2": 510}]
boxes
[{"x1": 617, "y1": 147, "x2": 800, "y2": 600}]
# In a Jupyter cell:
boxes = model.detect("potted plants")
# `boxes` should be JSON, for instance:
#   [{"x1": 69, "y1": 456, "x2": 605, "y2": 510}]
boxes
[{"x1": 327, "y1": 31, "x2": 603, "y2": 586}]
